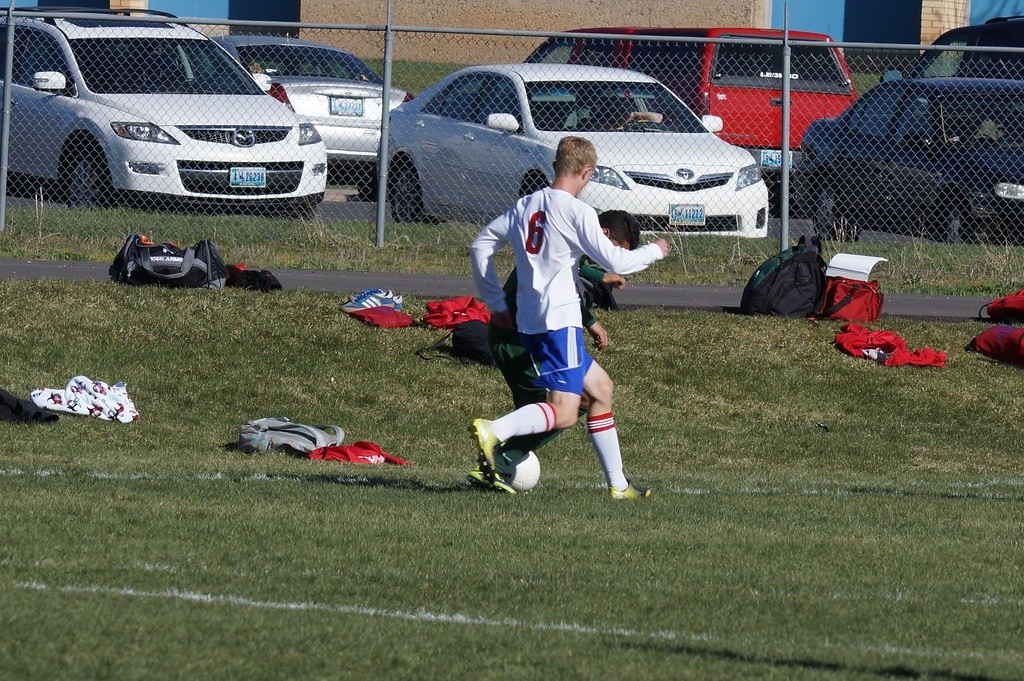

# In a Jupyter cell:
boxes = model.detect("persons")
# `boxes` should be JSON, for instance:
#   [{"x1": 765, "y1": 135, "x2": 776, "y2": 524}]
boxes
[
  {"x1": 467, "y1": 210, "x2": 640, "y2": 494},
  {"x1": 468, "y1": 137, "x2": 670, "y2": 502},
  {"x1": 609, "y1": 95, "x2": 663, "y2": 131}
]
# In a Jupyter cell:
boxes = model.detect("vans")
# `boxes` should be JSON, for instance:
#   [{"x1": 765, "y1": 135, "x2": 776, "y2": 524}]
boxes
[
  {"x1": 0, "y1": 6, "x2": 328, "y2": 215},
  {"x1": 520, "y1": 21, "x2": 856, "y2": 182},
  {"x1": 888, "y1": 13, "x2": 1023, "y2": 80}
]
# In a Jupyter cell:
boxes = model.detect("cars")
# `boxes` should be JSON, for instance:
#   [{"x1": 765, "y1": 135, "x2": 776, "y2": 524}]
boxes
[
  {"x1": 377, "y1": 60, "x2": 770, "y2": 239},
  {"x1": 799, "y1": 72, "x2": 1024, "y2": 243},
  {"x1": 209, "y1": 33, "x2": 414, "y2": 198}
]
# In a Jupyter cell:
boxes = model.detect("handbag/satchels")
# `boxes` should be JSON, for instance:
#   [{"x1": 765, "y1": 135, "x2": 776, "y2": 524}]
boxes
[
  {"x1": 818, "y1": 276, "x2": 884, "y2": 322},
  {"x1": 452, "y1": 320, "x2": 493, "y2": 360},
  {"x1": 109, "y1": 233, "x2": 228, "y2": 289},
  {"x1": 226, "y1": 264, "x2": 282, "y2": 292}
]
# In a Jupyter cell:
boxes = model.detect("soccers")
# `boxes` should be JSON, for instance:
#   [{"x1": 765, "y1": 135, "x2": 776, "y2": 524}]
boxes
[{"x1": 495, "y1": 445, "x2": 541, "y2": 492}]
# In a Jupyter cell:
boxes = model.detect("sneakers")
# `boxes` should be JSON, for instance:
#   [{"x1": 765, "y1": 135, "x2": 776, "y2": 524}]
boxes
[
  {"x1": 609, "y1": 480, "x2": 651, "y2": 500},
  {"x1": 473, "y1": 418, "x2": 503, "y2": 480},
  {"x1": 340, "y1": 288, "x2": 402, "y2": 312},
  {"x1": 467, "y1": 466, "x2": 516, "y2": 494}
]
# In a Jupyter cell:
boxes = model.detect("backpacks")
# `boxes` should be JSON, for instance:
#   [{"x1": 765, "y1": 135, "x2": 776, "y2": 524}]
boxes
[
  {"x1": 740, "y1": 235, "x2": 827, "y2": 316},
  {"x1": 239, "y1": 416, "x2": 345, "y2": 454}
]
